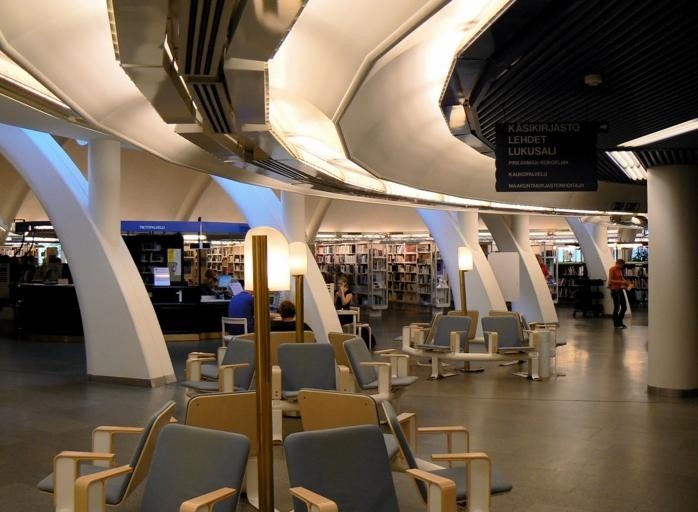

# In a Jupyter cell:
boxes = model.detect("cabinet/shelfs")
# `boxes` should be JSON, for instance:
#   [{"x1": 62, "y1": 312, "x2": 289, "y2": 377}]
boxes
[
  {"x1": 316, "y1": 238, "x2": 452, "y2": 317},
  {"x1": 124, "y1": 234, "x2": 244, "y2": 290},
  {"x1": 620, "y1": 261, "x2": 647, "y2": 308},
  {"x1": 528, "y1": 241, "x2": 586, "y2": 305}
]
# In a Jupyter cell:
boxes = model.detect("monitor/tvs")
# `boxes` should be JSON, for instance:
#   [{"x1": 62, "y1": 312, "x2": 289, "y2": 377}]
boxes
[{"x1": 217, "y1": 274, "x2": 232, "y2": 289}]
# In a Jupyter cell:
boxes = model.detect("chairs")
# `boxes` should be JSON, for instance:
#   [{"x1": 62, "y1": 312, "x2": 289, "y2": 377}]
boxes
[
  {"x1": 73, "y1": 424, "x2": 252, "y2": 512},
  {"x1": 34, "y1": 396, "x2": 177, "y2": 504},
  {"x1": 178, "y1": 311, "x2": 419, "y2": 420},
  {"x1": 168, "y1": 386, "x2": 259, "y2": 464},
  {"x1": 296, "y1": 384, "x2": 419, "y2": 459},
  {"x1": 281, "y1": 423, "x2": 459, "y2": 512},
  {"x1": 396, "y1": 310, "x2": 566, "y2": 383},
  {"x1": 379, "y1": 396, "x2": 515, "y2": 512}
]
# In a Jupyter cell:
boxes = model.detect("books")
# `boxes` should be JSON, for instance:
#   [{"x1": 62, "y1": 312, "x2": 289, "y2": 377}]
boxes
[
  {"x1": 184, "y1": 245, "x2": 243, "y2": 284},
  {"x1": 620, "y1": 264, "x2": 648, "y2": 308},
  {"x1": 556, "y1": 263, "x2": 585, "y2": 297},
  {"x1": 315, "y1": 242, "x2": 440, "y2": 306}
]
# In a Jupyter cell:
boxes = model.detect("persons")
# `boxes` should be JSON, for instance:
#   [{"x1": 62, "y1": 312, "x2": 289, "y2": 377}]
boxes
[
  {"x1": 186, "y1": 268, "x2": 237, "y2": 300},
  {"x1": 0, "y1": 252, "x2": 74, "y2": 313},
  {"x1": 228, "y1": 290, "x2": 255, "y2": 335},
  {"x1": 333, "y1": 275, "x2": 353, "y2": 327},
  {"x1": 268, "y1": 300, "x2": 317, "y2": 342},
  {"x1": 534, "y1": 253, "x2": 550, "y2": 280},
  {"x1": 608, "y1": 259, "x2": 629, "y2": 329}
]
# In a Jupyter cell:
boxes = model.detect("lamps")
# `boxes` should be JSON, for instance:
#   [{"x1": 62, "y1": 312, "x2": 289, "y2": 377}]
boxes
[
  {"x1": 458, "y1": 245, "x2": 484, "y2": 372},
  {"x1": 244, "y1": 226, "x2": 289, "y2": 512},
  {"x1": 289, "y1": 241, "x2": 307, "y2": 344}
]
